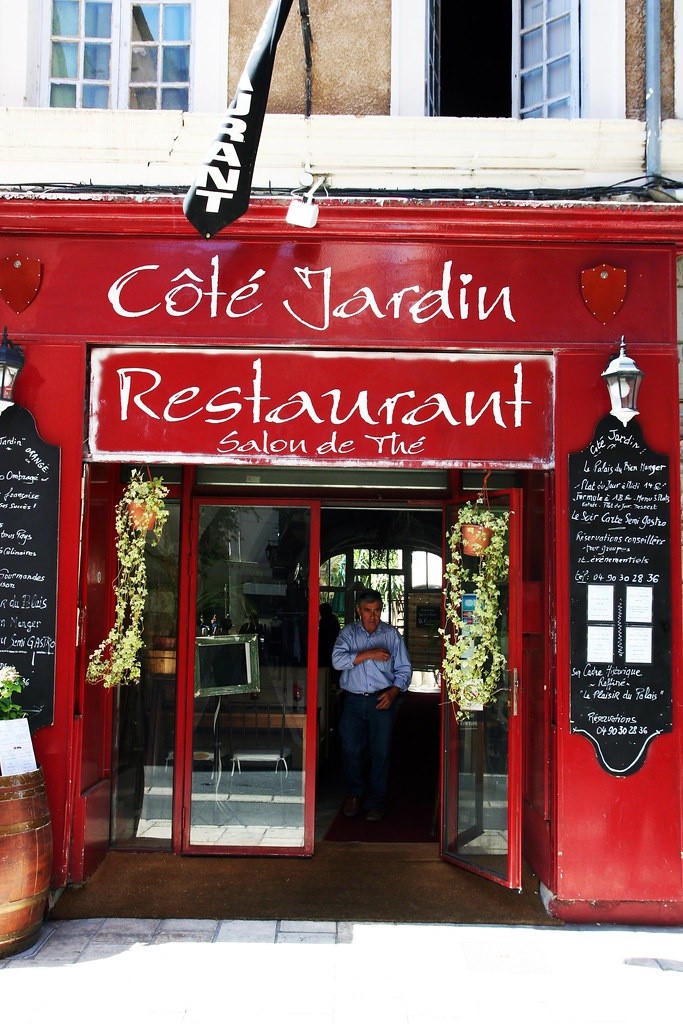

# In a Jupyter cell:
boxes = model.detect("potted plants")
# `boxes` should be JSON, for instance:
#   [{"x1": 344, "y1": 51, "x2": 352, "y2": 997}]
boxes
[
  {"x1": 83, "y1": 476, "x2": 171, "y2": 694},
  {"x1": 437, "y1": 499, "x2": 515, "y2": 726}
]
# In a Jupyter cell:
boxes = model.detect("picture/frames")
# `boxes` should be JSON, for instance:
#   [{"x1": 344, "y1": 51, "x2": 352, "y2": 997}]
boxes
[{"x1": 193, "y1": 632, "x2": 262, "y2": 699}]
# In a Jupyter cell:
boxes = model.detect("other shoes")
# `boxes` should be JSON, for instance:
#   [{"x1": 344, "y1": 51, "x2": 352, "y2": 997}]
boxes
[
  {"x1": 343, "y1": 797, "x2": 360, "y2": 816},
  {"x1": 367, "y1": 809, "x2": 386, "y2": 821}
]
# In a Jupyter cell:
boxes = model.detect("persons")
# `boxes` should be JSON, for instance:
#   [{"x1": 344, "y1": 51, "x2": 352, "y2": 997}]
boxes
[
  {"x1": 318, "y1": 601, "x2": 343, "y2": 718},
  {"x1": 331, "y1": 590, "x2": 413, "y2": 821}
]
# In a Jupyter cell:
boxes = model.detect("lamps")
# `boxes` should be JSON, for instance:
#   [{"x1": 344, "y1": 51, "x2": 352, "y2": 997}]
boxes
[
  {"x1": 285, "y1": 174, "x2": 326, "y2": 229},
  {"x1": 0, "y1": 324, "x2": 26, "y2": 416},
  {"x1": 601, "y1": 332, "x2": 646, "y2": 428}
]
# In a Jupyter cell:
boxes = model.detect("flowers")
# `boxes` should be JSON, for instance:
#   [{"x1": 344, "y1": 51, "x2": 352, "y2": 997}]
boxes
[{"x1": 0, "y1": 665, "x2": 29, "y2": 720}]
